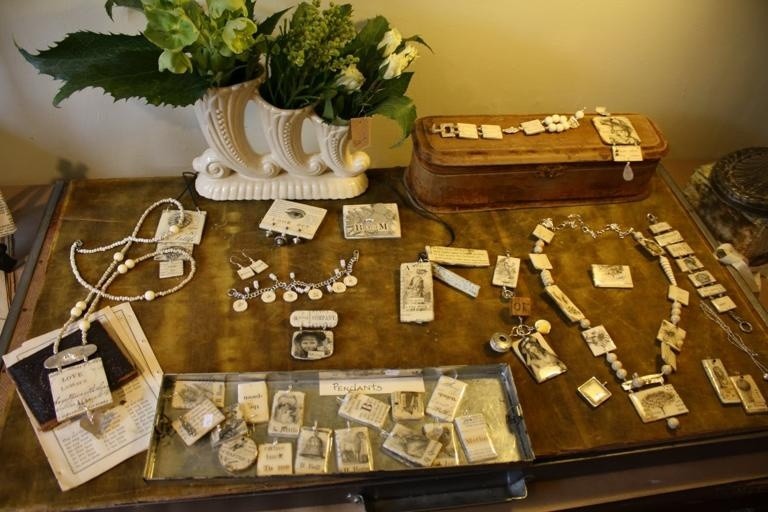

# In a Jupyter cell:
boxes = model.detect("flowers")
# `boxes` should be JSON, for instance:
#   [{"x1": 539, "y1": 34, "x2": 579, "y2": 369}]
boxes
[{"x1": 11, "y1": 0, "x2": 436, "y2": 150}]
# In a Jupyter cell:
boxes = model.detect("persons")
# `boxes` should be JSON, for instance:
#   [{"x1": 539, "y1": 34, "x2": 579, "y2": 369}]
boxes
[{"x1": 293, "y1": 331, "x2": 327, "y2": 358}]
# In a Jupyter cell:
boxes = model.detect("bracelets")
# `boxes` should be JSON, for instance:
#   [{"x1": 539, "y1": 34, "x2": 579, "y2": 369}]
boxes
[
  {"x1": 227, "y1": 250, "x2": 361, "y2": 313},
  {"x1": 647, "y1": 213, "x2": 753, "y2": 333},
  {"x1": 426, "y1": 106, "x2": 588, "y2": 141}
]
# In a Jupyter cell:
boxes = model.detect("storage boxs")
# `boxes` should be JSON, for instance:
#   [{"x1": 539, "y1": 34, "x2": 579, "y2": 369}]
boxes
[{"x1": 400, "y1": 111, "x2": 672, "y2": 216}]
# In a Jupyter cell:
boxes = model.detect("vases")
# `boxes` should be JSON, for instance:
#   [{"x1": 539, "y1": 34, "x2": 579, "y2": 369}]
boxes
[{"x1": 191, "y1": 63, "x2": 372, "y2": 201}]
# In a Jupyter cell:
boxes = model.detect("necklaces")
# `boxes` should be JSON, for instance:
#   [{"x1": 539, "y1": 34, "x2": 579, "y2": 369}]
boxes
[
  {"x1": 530, "y1": 213, "x2": 688, "y2": 430},
  {"x1": 701, "y1": 304, "x2": 768, "y2": 384},
  {"x1": 40, "y1": 197, "x2": 196, "y2": 424}
]
